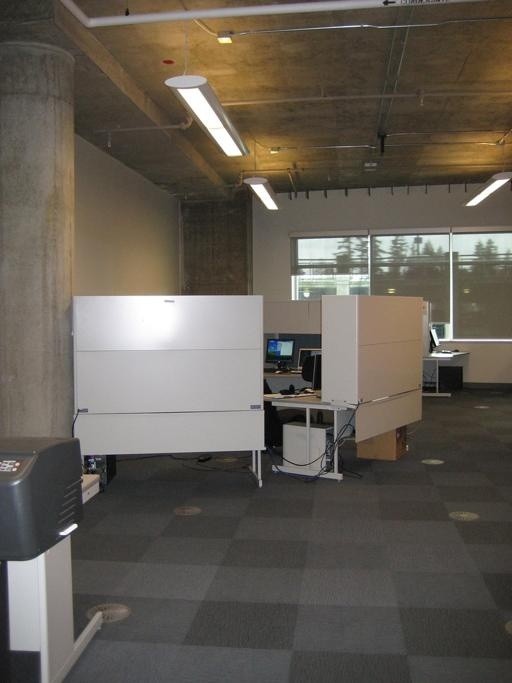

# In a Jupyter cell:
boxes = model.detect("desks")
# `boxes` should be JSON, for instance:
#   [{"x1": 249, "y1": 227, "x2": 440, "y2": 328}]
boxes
[
  {"x1": 263, "y1": 371, "x2": 348, "y2": 481},
  {"x1": 422, "y1": 351, "x2": 470, "y2": 397}
]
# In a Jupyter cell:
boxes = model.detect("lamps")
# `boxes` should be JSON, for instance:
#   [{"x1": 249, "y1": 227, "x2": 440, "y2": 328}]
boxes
[
  {"x1": 243, "y1": 130, "x2": 281, "y2": 211},
  {"x1": 462, "y1": 143, "x2": 512, "y2": 207},
  {"x1": 164, "y1": 20, "x2": 250, "y2": 158}
]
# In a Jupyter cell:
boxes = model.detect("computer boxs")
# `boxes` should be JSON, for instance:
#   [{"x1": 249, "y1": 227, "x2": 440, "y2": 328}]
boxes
[
  {"x1": 439, "y1": 367, "x2": 462, "y2": 389},
  {"x1": 282, "y1": 421, "x2": 332, "y2": 478}
]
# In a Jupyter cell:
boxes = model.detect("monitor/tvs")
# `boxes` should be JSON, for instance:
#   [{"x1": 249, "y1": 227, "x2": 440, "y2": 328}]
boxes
[
  {"x1": 429, "y1": 326, "x2": 437, "y2": 355},
  {"x1": 266, "y1": 338, "x2": 295, "y2": 376}
]
